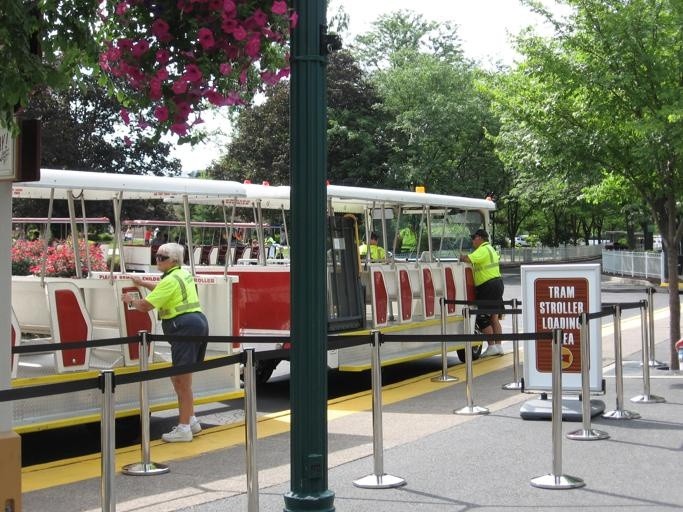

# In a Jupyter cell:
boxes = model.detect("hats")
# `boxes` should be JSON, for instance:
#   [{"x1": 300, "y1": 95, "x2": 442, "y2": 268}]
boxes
[{"x1": 469, "y1": 229, "x2": 489, "y2": 239}]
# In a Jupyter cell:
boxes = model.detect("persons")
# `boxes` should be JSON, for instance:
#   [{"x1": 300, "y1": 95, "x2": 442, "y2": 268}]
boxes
[
  {"x1": 359, "y1": 232, "x2": 384, "y2": 260},
  {"x1": 460, "y1": 229, "x2": 504, "y2": 358},
  {"x1": 397, "y1": 220, "x2": 423, "y2": 253},
  {"x1": 121, "y1": 242, "x2": 209, "y2": 443},
  {"x1": 617, "y1": 235, "x2": 629, "y2": 247},
  {"x1": 246, "y1": 239, "x2": 259, "y2": 247},
  {"x1": 264, "y1": 234, "x2": 274, "y2": 247},
  {"x1": 121, "y1": 227, "x2": 133, "y2": 246},
  {"x1": 144, "y1": 226, "x2": 183, "y2": 246}
]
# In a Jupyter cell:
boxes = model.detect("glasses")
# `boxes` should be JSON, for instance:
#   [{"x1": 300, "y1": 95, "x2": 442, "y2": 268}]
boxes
[{"x1": 155, "y1": 254, "x2": 173, "y2": 261}]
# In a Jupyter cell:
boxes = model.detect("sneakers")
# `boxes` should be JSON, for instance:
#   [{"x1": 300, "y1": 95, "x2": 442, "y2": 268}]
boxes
[
  {"x1": 191, "y1": 421, "x2": 202, "y2": 434},
  {"x1": 479, "y1": 344, "x2": 504, "y2": 357},
  {"x1": 161, "y1": 426, "x2": 193, "y2": 442}
]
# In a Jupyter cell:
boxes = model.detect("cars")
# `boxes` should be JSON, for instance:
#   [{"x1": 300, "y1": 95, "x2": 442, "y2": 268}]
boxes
[{"x1": 509, "y1": 235, "x2": 541, "y2": 246}]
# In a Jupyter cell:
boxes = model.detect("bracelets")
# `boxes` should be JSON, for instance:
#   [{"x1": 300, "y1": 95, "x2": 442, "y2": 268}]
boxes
[{"x1": 130, "y1": 300, "x2": 133, "y2": 305}]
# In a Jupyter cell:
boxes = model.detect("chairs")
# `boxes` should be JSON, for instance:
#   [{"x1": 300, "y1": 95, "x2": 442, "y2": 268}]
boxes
[
  {"x1": 225, "y1": 247, "x2": 236, "y2": 266},
  {"x1": 242, "y1": 248, "x2": 252, "y2": 266},
  {"x1": 124, "y1": 243, "x2": 152, "y2": 275},
  {"x1": 258, "y1": 247, "x2": 269, "y2": 265},
  {"x1": 207, "y1": 247, "x2": 219, "y2": 266},
  {"x1": 191, "y1": 246, "x2": 202, "y2": 266}
]
